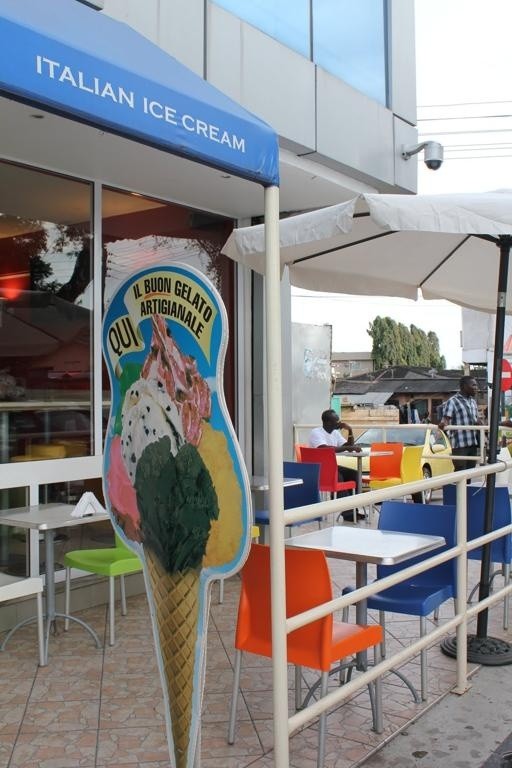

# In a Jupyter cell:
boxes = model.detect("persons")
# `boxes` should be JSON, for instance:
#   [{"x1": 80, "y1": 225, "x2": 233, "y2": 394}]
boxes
[
  {"x1": 438, "y1": 375, "x2": 484, "y2": 484},
  {"x1": 308, "y1": 408, "x2": 369, "y2": 522},
  {"x1": 422, "y1": 411, "x2": 432, "y2": 424}
]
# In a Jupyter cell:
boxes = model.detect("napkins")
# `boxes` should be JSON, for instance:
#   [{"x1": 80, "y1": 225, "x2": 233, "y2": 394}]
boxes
[{"x1": 70, "y1": 491, "x2": 108, "y2": 518}]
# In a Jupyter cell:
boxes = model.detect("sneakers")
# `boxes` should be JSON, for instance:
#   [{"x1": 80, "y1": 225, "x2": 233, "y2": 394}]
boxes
[{"x1": 343, "y1": 511, "x2": 365, "y2": 522}]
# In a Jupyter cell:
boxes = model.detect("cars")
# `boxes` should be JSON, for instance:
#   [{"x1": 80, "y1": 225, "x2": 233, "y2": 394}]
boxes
[{"x1": 335, "y1": 424, "x2": 454, "y2": 504}]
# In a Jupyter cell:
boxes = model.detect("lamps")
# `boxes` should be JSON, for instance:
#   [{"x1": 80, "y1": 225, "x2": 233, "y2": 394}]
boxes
[{"x1": 401, "y1": 139, "x2": 443, "y2": 169}]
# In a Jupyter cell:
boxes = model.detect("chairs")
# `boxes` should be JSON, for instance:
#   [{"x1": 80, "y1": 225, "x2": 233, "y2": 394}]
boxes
[
  {"x1": 63, "y1": 532, "x2": 146, "y2": 647},
  {"x1": 1, "y1": 572, "x2": 47, "y2": 668},
  {"x1": 255, "y1": 461, "x2": 322, "y2": 546},
  {"x1": 226, "y1": 542, "x2": 385, "y2": 767},
  {"x1": 219, "y1": 523, "x2": 260, "y2": 604},
  {"x1": 299, "y1": 446, "x2": 357, "y2": 525},
  {"x1": 442, "y1": 484, "x2": 511, "y2": 631},
  {"x1": 368, "y1": 447, "x2": 424, "y2": 525},
  {"x1": 339, "y1": 500, "x2": 459, "y2": 700},
  {"x1": 362, "y1": 442, "x2": 403, "y2": 513},
  {"x1": 295, "y1": 444, "x2": 307, "y2": 463}
]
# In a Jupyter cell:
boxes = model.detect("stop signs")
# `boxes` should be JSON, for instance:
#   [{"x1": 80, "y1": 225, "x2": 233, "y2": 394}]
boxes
[{"x1": 501, "y1": 359, "x2": 512, "y2": 392}]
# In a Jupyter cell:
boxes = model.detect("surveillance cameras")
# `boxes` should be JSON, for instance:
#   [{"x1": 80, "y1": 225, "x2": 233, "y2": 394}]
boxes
[{"x1": 424, "y1": 143, "x2": 443, "y2": 170}]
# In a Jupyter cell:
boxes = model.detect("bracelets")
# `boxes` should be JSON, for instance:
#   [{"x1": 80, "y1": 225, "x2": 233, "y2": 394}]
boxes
[{"x1": 348, "y1": 434, "x2": 354, "y2": 437}]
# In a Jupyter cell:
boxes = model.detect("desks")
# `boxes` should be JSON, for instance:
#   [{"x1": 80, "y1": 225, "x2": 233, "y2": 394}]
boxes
[
  {"x1": 249, "y1": 475, "x2": 304, "y2": 544},
  {"x1": 336, "y1": 452, "x2": 394, "y2": 518},
  {"x1": 283, "y1": 525, "x2": 448, "y2": 734},
  {"x1": 1, "y1": 502, "x2": 113, "y2": 667}
]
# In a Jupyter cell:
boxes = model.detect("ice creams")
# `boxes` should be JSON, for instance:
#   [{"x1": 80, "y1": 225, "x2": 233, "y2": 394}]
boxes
[{"x1": 119, "y1": 314, "x2": 219, "y2": 768}]
